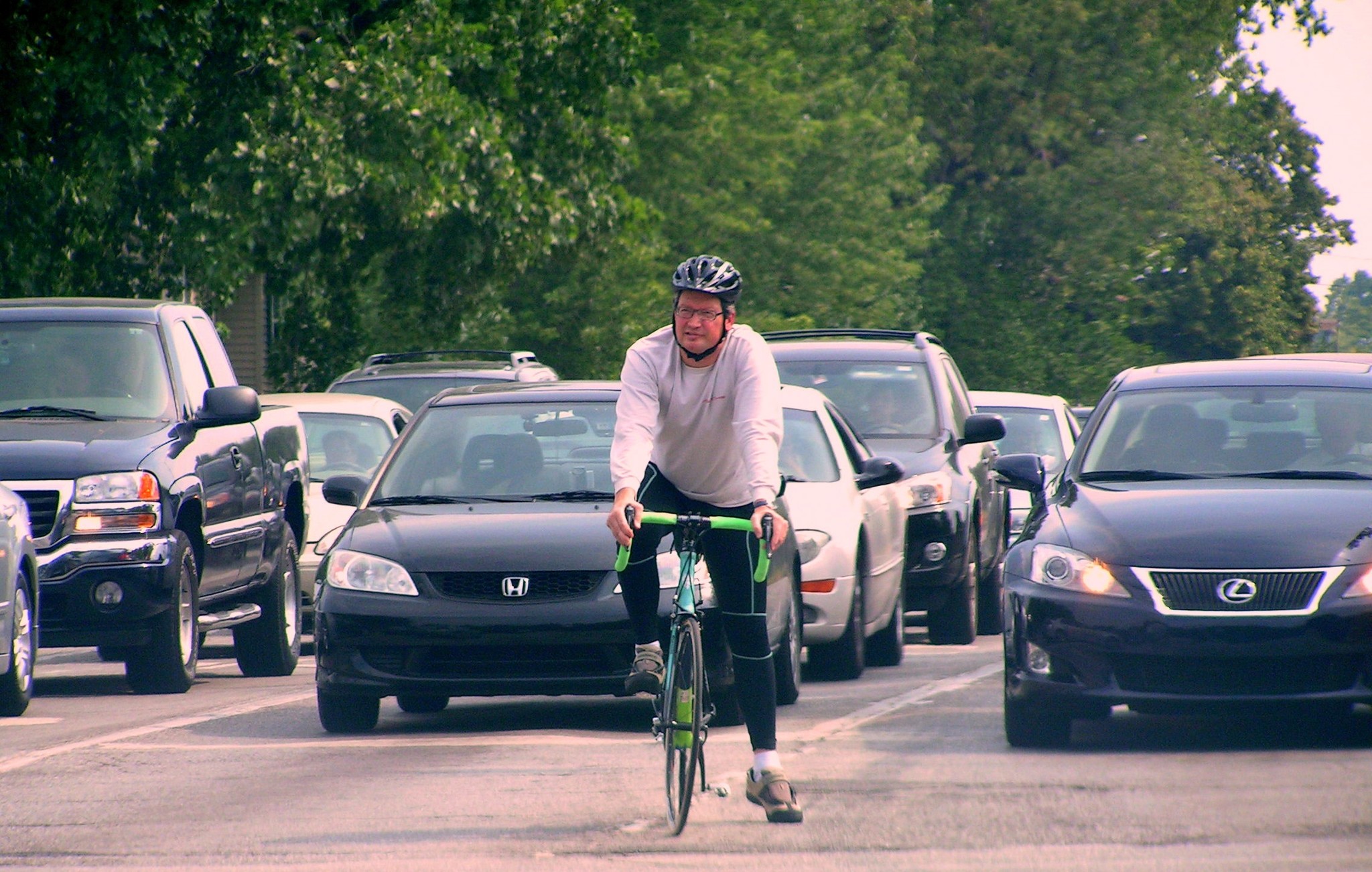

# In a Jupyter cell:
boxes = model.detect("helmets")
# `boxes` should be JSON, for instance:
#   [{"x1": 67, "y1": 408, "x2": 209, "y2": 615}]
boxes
[{"x1": 673, "y1": 254, "x2": 743, "y2": 302}]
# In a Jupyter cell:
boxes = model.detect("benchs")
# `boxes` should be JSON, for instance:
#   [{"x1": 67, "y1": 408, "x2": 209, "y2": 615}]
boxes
[{"x1": 1225, "y1": 431, "x2": 1320, "y2": 471}]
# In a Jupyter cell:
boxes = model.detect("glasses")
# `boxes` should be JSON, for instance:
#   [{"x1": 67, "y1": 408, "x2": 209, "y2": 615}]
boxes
[{"x1": 675, "y1": 305, "x2": 724, "y2": 321}]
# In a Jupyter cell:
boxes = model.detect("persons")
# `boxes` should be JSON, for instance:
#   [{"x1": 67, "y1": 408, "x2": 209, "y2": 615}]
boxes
[
  {"x1": 1287, "y1": 389, "x2": 1372, "y2": 476},
  {"x1": 607, "y1": 253, "x2": 806, "y2": 823},
  {"x1": 321, "y1": 429, "x2": 360, "y2": 470}
]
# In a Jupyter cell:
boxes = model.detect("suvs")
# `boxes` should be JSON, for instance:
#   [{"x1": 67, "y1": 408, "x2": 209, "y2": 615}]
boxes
[
  {"x1": 754, "y1": 330, "x2": 1014, "y2": 647},
  {"x1": 318, "y1": 351, "x2": 564, "y2": 457}
]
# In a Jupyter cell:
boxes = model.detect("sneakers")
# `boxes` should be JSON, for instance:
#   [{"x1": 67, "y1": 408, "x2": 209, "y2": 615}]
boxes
[
  {"x1": 624, "y1": 645, "x2": 666, "y2": 694},
  {"x1": 746, "y1": 768, "x2": 803, "y2": 823}
]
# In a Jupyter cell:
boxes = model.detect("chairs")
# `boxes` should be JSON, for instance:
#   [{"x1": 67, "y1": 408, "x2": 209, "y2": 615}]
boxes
[
  {"x1": 1133, "y1": 405, "x2": 1228, "y2": 473},
  {"x1": 462, "y1": 433, "x2": 565, "y2": 494}
]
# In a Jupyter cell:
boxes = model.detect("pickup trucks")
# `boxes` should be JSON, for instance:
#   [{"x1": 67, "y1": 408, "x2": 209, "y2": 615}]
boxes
[{"x1": 1, "y1": 294, "x2": 307, "y2": 695}]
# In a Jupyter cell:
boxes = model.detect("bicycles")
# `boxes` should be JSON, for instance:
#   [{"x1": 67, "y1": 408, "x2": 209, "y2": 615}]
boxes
[{"x1": 613, "y1": 501, "x2": 779, "y2": 834}]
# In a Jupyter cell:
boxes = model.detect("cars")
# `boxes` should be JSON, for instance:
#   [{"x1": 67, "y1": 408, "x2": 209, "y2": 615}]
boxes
[
  {"x1": 774, "y1": 385, "x2": 913, "y2": 678},
  {"x1": 248, "y1": 390, "x2": 418, "y2": 632},
  {"x1": 0, "y1": 482, "x2": 42, "y2": 718},
  {"x1": 955, "y1": 351, "x2": 1372, "y2": 745},
  {"x1": 312, "y1": 383, "x2": 809, "y2": 736}
]
